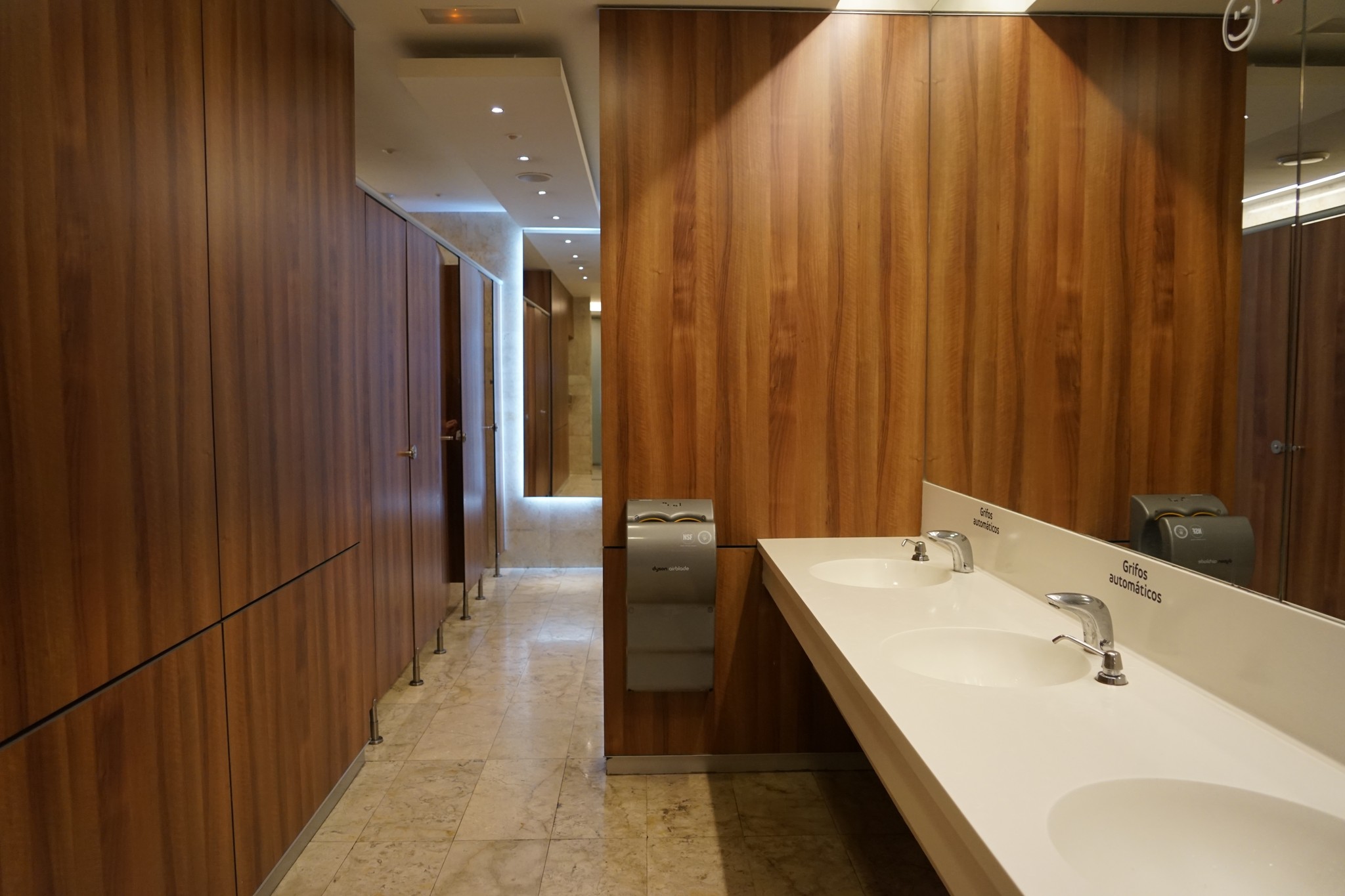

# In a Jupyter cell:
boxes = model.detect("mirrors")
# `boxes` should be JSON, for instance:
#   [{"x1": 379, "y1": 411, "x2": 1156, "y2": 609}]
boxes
[
  {"x1": 523, "y1": 229, "x2": 602, "y2": 497},
  {"x1": 925, "y1": 2, "x2": 1345, "y2": 624}
]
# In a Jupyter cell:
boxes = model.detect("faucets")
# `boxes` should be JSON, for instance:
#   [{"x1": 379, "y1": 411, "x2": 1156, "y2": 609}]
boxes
[
  {"x1": 925, "y1": 530, "x2": 974, "y2": 573},
  {"x1": 1046, "y1": 593, "x2": 1115, "y2": 657}
]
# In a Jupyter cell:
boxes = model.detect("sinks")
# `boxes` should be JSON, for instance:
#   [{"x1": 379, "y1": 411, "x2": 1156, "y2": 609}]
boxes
[
  {"x1": 1047, "y1": 776, "x2": 1344, "y2": 896},
  {"x1": 808, "y1": 558, "x2": 953, "y2": 589},
  {"x1": 879, "y1": 627, "x2": 1094, "y2": 688}
]
